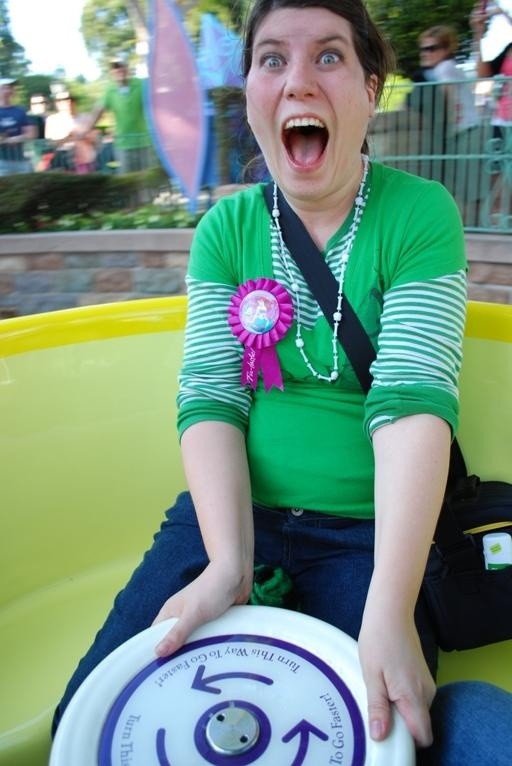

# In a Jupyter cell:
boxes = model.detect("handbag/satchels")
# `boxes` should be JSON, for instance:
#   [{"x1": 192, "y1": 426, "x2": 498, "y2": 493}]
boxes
[{"x1": 423, "y1": 474, "x2": 512, "y2": 653}]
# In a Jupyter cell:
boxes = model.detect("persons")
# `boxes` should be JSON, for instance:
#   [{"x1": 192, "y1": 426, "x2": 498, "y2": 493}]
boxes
[{"x1": 50, "y1": 1, "x2": 470, "y2": 766}]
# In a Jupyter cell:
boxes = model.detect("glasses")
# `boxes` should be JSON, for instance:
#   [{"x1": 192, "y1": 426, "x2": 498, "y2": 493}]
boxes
[{"x1": 418, "y1": 43, "x2": 447, "y2": 55}]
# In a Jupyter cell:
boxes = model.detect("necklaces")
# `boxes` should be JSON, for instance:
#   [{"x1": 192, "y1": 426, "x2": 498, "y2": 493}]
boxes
[{"x1": 272, "y1": 151, "x2": 369, "y2": 382}]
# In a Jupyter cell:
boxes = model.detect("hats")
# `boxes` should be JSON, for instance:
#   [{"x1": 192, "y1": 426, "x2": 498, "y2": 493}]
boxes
[{"x1": 1, "y1": 76, "x2": 18, "y2": 89}]
